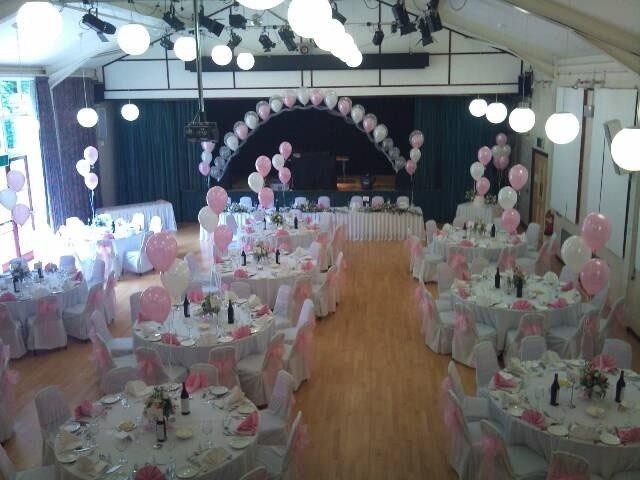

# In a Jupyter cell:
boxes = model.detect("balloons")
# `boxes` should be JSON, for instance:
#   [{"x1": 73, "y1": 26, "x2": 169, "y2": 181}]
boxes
[
  {"x1": 196, "y1": 87, "x2": 425, "y2": 192},
  {"x1": 508, "y1": 163, "x2": 529, "y2": 192},
  {"x1": 502, "y1": 208, "x2": 520, "y2": 233},
  {"x1": 198, "y1": 184, "x2": 235, "y2": 254},
  {"x1": 0, "y1": 170, "x2": 31, "y2": 230},
  {"x1": 139, "y1": 284, "x2": 171, "y2": 325},
  {"x1": 470, "y1": 134, "x2": 512, "y2": 195},
  {"x1": 159, "y1": 259, "x2": 192, "y2": 299},
  {"x1": 582, "y1": 210, "x2": 611, "y2": 251},
  {"x1": 559, "y1": 235, "x2": 593, "y2": 274},
  {"x1": 145, "y1": 232, "x2": 179, "y2": 275},
  {"x1": 579, "y1": 256, "x2": 610, "y2": 298},
  {"x1": 258, "y1": 187, "x2": 274, "y2": 208},
  {"x1": 498, "y1": 187, "x2": 517, "y2": 210},
  {"x1": 74, "y1": 145, "x2": 99, "y2": 192}
]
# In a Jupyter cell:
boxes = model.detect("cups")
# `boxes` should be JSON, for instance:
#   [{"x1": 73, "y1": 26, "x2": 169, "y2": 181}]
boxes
[{"x1": 127, "y1": 226, "x2": 136, "y2": 236}]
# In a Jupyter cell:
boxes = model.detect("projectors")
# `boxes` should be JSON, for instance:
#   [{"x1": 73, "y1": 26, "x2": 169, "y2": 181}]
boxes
[{"x1": 183, "y1": 122, "x2": 219, "y2": 138}]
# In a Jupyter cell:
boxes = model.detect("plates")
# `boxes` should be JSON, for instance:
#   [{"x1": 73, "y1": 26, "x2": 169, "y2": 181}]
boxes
[
  {"x1": 586, "y1": 405, "x2": 605, "y2": 417},
  {"x1": 436, "y1": 218, "x2": 527, "y2": 250},
  {"x1": 459, "y1": 266, "x2": 578, "y2": 311},
  {"x1": 175, "y1": 386, "x2": 256, "y2": 478},
  {"x1": 137, "y1": 293, "x2": 267, "y2": 347},
  {"x1": 570, "y1": 360, "x2": 589, "y2": 367},
  {"x1": 547, "y1": 425, "x2": 569, "y2": 437},
  {"x1": 55, "y1": 393, "x2": 142, "y2": 480},
  {"x1": 216, "y1": 246, "x2": 313, "y2": 279},
  {"x1": 600, "y1": 433, "x2": 621, "y2": 445},
  {"x1": 149, "y1": 454, "x2": 175, "y2": 465},
  {"x1": 618, "y1": 369, "x2": 638, "y2": 378},
  {"x1": 158, "y1": 383, "x2": 179, "y2": 390},
  {"x1": 496, "y1": 361, "x2": 547, "y2": 416}
]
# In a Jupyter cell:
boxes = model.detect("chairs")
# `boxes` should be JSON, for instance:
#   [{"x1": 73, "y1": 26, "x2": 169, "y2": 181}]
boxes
[{"x1": 198, "y1": 195, "x2": 424, "y2": 209}]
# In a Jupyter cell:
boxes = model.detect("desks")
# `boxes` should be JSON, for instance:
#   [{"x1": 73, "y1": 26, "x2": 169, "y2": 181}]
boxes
[
  {"x1": 94, "y1": 199, "x2": 177, "y2": 233},
  {"x1": 199, "y1": 204, "x2": 425, "y2": 242}
]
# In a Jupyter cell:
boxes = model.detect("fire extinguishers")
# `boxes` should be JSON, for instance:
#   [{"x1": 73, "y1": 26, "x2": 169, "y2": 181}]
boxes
[{"x1": 544, "y1": 210, "x2": 554, "y2": 235}]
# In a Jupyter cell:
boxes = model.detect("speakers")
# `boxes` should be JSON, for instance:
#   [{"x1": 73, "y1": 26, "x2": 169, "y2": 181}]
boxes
[
  {"x1": 94, "y1": 83, "x2": 104, "y2": 104},
  {"x1": 519, "y1": 72, "x2": 533, "y2": 97}
]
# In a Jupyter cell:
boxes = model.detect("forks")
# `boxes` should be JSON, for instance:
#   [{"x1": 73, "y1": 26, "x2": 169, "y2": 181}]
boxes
[{"x1": 563, "y1": 361, "x2": 573, "y2": 370}]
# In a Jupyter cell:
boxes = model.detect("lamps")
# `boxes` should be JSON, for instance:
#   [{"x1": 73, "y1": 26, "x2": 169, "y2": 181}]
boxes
[
  {"x1": 121, "y1": 56, "x2": 141, "y2": 123},
  {"x1": 609, "y1": 124, "x2": 640, "y2": 173},
  {"x1": 467, "y1": 14, "x2": 580, "y2": 146},
  {"x1": 75, "y1": 30, "x2": 99, "y2": 129}
]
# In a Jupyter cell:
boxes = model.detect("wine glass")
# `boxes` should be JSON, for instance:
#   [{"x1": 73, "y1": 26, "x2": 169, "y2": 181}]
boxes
[
  {"x1": 151, "y1": 432, "x2": 161, "y2": 449},
  {"x1": 0, "y1": 255, "x2": 80, "y2": 300}
]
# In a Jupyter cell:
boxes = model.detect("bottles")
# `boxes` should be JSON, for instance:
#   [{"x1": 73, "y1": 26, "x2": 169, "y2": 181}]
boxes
[
  {"x1": 262, "y1": 216, "x2": 266, "y2": 230},
  {"x1": 88, "y1": 218, "x2": 91, "y2": 225},
  {"x1": 294, "y1": 214, "x2": 298, "y2": 229},
  {"x1": 179, "y1": 382, "x2": 190, "y2": 415},
  {"x1": 112, "y1": 221, "x2": 115, "y2": 233},
  {"x1": 615, "y1": 370, "x2": 626, "y2": 402},
  {"x1": 549, "y1": 372, "x2": 561, "y2": 405},
  {"x1": 156, "y1": 408, "x2": 167, "y2": 442}
]
